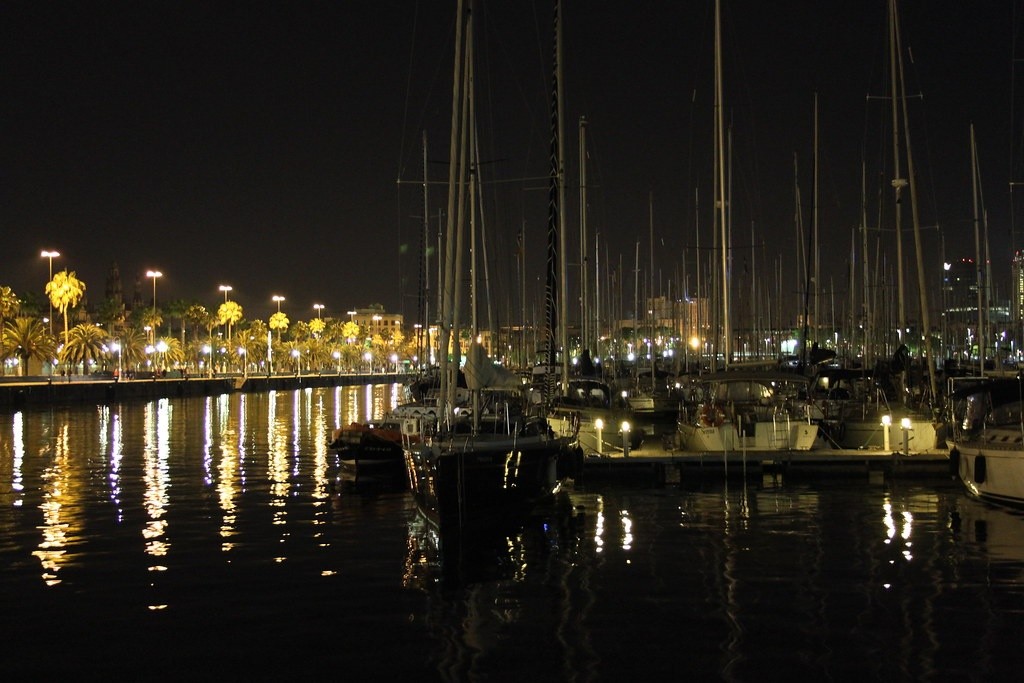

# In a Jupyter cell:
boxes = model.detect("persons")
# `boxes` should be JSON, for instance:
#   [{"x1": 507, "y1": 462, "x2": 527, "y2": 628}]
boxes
[
  {"x1": 157, "y1": 367, "x2": 167, "y2": 378},
  {"x1": 891, "y1": 345, "x2": 908, "y2": 371},
  {"x1": 60, "y1": 369, "x2": 73, "y2": 382},
  {"x1": 582, "y1": 352, "x2": 595, "y2": 376},
  {"x1": 180, "y1": 367, "x2": 191, "y2": 378},
  {"x1": 113, "y1": 368, "x2": 133, "y2": 383},
  {"x1": 201, "y1": 368, "x2": 217, "y2": 378}
]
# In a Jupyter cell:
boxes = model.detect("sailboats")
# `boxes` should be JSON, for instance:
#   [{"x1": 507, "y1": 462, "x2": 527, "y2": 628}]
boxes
[{"x1": 329, "y1": 0, "x2": 1023, "y2": 555}]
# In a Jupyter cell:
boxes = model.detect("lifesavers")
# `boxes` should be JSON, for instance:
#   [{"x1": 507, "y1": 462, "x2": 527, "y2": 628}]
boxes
[
  {"x1": 975, "y1": 520, "x2": 988, "y2": 543},
  {"x1": 949, "y1": 450, "x2": 960, "y2": 474},
  {"x1": 974, "y1": 456, "x2": 986, "y2": 483},
  {"x1": 572, "y1": 411, "x2": 581, "y2": 435},
  {"x1": 951, "y1": 511, "x2": 961, "y2": 533},
  {"x1": 701, "y1": 404, "x2": 723, "y2": 426}
]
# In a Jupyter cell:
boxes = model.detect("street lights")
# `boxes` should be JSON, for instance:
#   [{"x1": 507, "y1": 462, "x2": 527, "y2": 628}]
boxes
[
  {"x1": 148, "y1": 271, "x2": 162, "y2": 379},
  {"x1": 365, "y1": 354, "x2": 372, "y2": 374},
  {"x1": 239, "y1": 348, "x2": 248, "y2": 379},
  {"x1": 313, "y1": 303, "x2": 324, "y2": 375},
  {"x1": 273, "y1": 296, "x2": 285, "y2": 376},
  {"x1": 334, "y1": 351, "x2": 341, "y2": 372},
  {"x1": 204, "y1": 346, "x2": 212, "y2": 379},
  {"x1": 373, "y1": 316, "x2": 381, "y2": 335},
  {"x1": 293, "y1": 350, "x2": 300, "y2": 376},
  {"x1": 220, "y1": 286, "x2": 232, "y2": 378},
  {"x1": 42, "y1": 251, "x2": 60, "y2": 375},
  {"x1": 391, "y1": 355, "x2": 397, "y2": 374},
  {"x1": 112, "y1": 336, "x2": 122, "y2": 382},
  {"x1": 347, "y1": 311, "x2": 357, "y2": 322}
]
